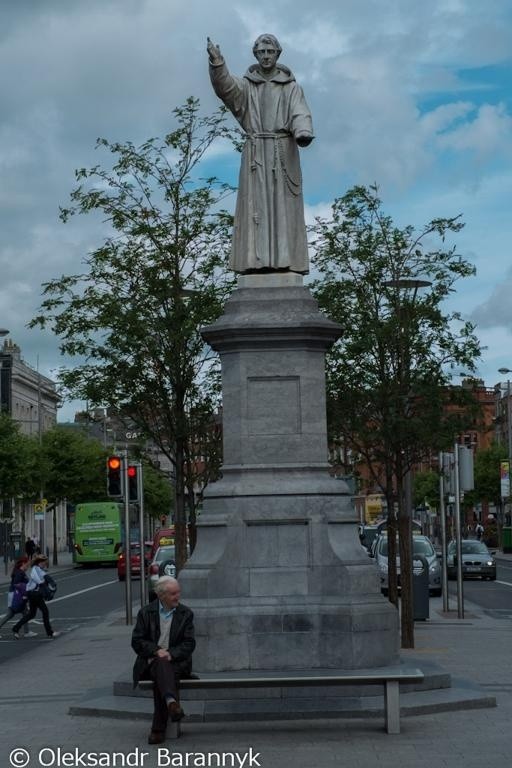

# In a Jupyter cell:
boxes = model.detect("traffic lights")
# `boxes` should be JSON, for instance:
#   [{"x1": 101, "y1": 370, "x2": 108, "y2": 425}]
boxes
[
  {"x1": 106, "y1": 456, "x2": 124, "y2": 498},
  {"x1": 128, "y1": 464, "x2": 139, "y2": 503}
]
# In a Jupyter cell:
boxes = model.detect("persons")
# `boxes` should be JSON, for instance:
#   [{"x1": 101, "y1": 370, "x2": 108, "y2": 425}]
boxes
[
  {"x1": 0, "y1": 534, "x2": 61, "y2": 640},
  {"x1": 131, "y1": 575, "x2": 196, "y2": 745},
  {"x1": 475, "y1": 522, "x2": 485, "y2": 540},
  {"x1": 206, "y1": 34, "x2": 314, "y2": 275}
]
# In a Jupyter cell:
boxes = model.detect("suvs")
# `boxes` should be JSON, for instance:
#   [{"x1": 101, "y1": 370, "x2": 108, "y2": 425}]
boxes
[{"x1": 147, "y1": 525, "x2": 190, "y2": 602}]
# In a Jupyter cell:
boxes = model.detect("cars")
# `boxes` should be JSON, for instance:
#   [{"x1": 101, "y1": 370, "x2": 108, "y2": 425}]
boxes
[
  {"x1": 359, "y1": 519, "x2": 444, "y2": 597},
  {"x1": 118, "y1": 541, "x2": 154, "y2": 580},
  {"x1": 447, "y1": 540, "x2": 497, "y2": 580}
]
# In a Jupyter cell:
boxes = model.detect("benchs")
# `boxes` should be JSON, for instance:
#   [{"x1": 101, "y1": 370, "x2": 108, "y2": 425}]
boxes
[{"x1": 138, "y1": 668, "x2": 424, "y2": 740}]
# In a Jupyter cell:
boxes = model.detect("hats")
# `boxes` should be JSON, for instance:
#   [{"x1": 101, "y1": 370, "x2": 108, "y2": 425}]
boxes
[{"x1": 32, "y1": 554, "x2": 48, "y2": 561}]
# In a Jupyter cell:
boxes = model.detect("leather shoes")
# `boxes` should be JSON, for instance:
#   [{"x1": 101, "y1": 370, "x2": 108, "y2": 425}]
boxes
[
  {"x1": 148, "y1": 729, "x2": 165, "y2": 744},
  {"x1": 168, "y1": 702, "x2": 185, "y2": 723}
]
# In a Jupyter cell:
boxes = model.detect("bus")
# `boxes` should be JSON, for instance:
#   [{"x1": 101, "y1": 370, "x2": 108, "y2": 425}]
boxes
[
  {"x1": 359, "y1": 491, "x2": 400, "y2": 524},
  {"x1": 73, "y1": 502, "x2": 138, "y2": 566}
]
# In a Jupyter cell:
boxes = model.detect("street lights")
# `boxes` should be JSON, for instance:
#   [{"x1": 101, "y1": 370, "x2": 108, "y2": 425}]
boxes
[
  {"x1": 499, "y1": 368, "x2": 511, "y2": 374},
  {"x1": 36, "y1": 377, "x2": 81, "y2": 553},
  {"x1": 381, "y1": 267, "x2": 431, "y2": 395}
]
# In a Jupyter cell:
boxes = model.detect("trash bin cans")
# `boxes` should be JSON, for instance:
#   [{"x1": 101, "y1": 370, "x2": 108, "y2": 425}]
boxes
[{"x1": 411, "y1": 554, "x2": 430, "y2": 621}]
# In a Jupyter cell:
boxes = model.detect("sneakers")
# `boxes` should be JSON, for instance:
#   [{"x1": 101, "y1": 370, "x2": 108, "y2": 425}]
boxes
[
  {"x1": 13, "y1": 632, "x2": 19, "y2": 640},
  {"x1": 23, "y1": 631, "x2": 38, "y2": 638},
  {"x1": 48, "y1": 632, "x2": 62, "y2": 638}
]
